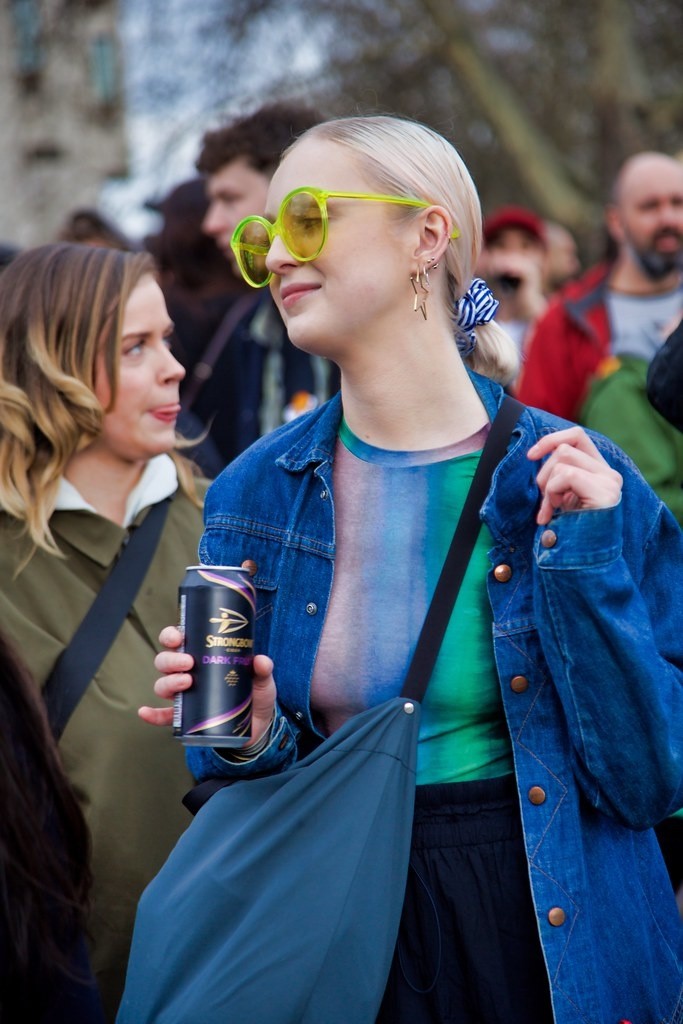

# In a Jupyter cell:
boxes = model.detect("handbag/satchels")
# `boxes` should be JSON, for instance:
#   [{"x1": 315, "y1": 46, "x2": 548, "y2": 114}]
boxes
[{"x1": 117, "y1": 695, "x2": 421, "y2": 1024}]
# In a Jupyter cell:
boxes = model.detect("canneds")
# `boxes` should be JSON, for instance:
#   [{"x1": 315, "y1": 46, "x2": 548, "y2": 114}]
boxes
[{"x1": 171, "y1": 565, "x2": 258, "y2": 748}]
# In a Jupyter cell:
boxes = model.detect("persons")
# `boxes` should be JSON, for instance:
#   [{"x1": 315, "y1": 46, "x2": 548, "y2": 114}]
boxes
[
  {"x1": 0, "y1": 94, "x2": 683, "y2": 1024},
  {"x1": 137, "y1": 116, "x2": 683, "y2": 1024}
]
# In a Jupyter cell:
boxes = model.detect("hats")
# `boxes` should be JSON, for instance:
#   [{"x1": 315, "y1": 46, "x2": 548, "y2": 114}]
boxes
[
  {"x1": 143, "y1": 180, "x2": 209, "y2": 217},
  {"x1": 482, "y1": 207, "x2": 544, "y2": 246}
]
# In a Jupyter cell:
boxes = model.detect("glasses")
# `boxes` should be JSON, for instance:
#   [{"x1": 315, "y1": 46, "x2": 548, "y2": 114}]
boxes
[{"x1": 229, "y1": 187, "x2": 458, "y2": 289}]
